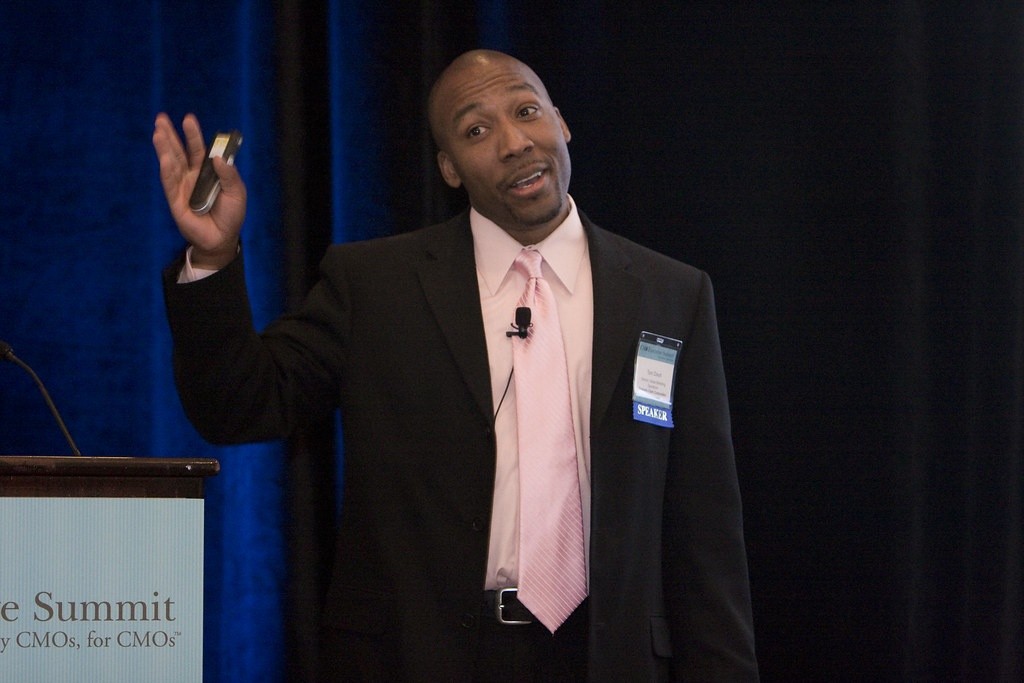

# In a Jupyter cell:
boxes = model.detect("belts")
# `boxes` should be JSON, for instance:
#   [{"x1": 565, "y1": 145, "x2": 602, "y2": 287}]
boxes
[{"x1": 475, "y1": 587, "x2": 588, "y2": 627}]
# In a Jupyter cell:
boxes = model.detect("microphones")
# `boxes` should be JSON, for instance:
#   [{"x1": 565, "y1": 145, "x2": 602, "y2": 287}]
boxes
[
  {"x1": 0, "y1": 341, "x2": 81, "y2": 457},
  {"x1": 514, "y1": 307, "x2": 531, "y2": 339}
]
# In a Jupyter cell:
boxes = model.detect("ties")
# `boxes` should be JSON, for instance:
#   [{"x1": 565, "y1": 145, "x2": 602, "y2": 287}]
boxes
[{"x1": 510, "y1": 250, "x2": 588, "y2": 635}]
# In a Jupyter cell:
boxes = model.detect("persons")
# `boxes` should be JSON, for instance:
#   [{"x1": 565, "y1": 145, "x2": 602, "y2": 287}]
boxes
[{"x1": 153, "y1": 50, "x2": 758, "y2": 683}]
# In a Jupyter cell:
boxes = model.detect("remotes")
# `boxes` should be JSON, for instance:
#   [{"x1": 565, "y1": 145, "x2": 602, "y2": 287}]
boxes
[{"x1": 189, "y1": 130, "x2": 245, "y2": 216}]
